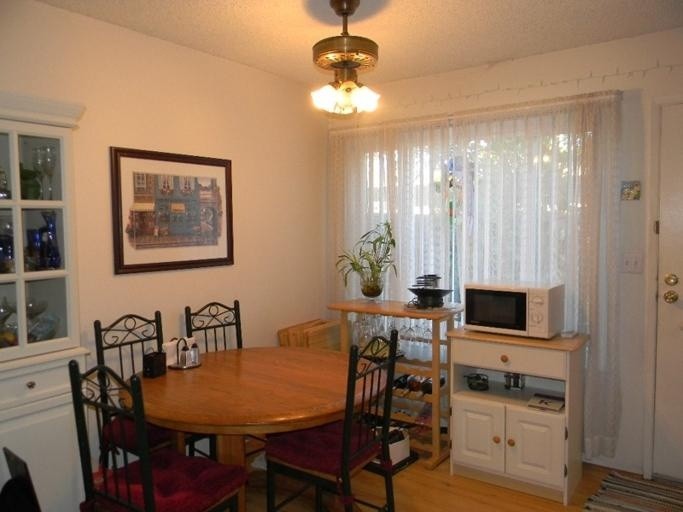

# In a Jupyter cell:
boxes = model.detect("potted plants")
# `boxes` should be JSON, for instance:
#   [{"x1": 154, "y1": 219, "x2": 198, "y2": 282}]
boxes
[{"x1": 333, "y1": 217, "x2": 399, "y2": 298}]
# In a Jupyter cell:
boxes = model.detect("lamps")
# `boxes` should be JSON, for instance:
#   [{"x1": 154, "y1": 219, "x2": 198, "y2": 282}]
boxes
[{"x1": 306, "y1": 2, "x2": 382, "y2": 116}]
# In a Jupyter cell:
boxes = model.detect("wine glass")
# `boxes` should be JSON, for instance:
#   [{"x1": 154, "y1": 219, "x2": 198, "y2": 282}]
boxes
[
  {"x1": 353, "y1": 314, "x2": 431, "y2": 352},
  {"x1": 31, "y1": 145, "x2": 56, "y2": 199},
  {"x1": 0, "y1": 280, "x2": 51, "y2": 351}
]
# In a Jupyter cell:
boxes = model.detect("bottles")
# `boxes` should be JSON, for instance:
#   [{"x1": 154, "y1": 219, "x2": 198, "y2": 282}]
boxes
[
  {"x1": 0, "y1": 212, "x2": 13, "y2": 274},
  {"x1": 189, "y1": 342, "x2": 199, "y2": 366},
  {"x1": 180, "y1": 346, "x2": 191, "y2": 369},
  {"x1": 393, "y1": 373, "x2": 445, "y2": 395}
]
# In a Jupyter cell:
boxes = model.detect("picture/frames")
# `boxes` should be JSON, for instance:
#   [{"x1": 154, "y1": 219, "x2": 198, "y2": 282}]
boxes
[{"x1": 110, "y1": 144, "x2": 234, "y2": 276}]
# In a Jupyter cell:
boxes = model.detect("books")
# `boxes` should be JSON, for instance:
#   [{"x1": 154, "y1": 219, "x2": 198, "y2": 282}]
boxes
[{"x1": 527, "y1": 392, "x2": 565, "y2": 412}]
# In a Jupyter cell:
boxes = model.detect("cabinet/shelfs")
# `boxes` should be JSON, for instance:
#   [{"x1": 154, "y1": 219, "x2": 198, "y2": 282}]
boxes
[
  {"x1": 0, "y1": 90, "x2": 92, "y2": 512},
  {"x1": 447, "y1": 333, "x2": 586, "y2": 506},
  {"x1": 327, "y1": 297, "x2": 464, "y2": 471}
]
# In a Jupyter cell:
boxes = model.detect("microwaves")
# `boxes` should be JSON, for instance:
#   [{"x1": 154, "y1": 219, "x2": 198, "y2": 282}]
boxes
[{"x1": 462, "y1": 281, "x2": 564, "y2": 340}]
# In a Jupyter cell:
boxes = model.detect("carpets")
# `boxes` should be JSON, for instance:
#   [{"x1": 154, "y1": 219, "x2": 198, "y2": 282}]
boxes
[{"x1": 582, "y1": 468, "x2": 683, "y2": 511}]
